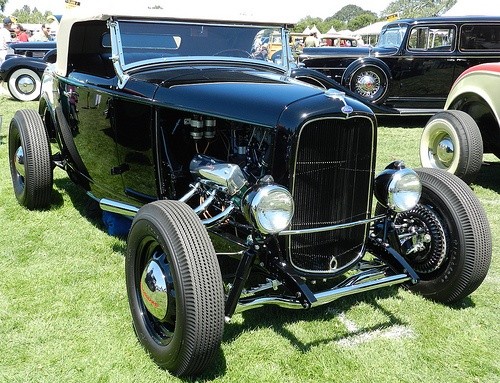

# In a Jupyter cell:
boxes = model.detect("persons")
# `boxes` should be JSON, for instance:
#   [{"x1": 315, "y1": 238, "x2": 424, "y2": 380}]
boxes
[
  {"x1": 302, "y1": 31, "x2": 321, "y2": 48},
  {"x1": 0, "y1": 16, "x2": 53, "y2": 66}
]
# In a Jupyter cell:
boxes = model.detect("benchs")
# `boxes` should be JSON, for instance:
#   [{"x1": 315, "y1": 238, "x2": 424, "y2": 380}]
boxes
[{"x1": 72, "y1": 49, "x2": 189, "y2": 78}]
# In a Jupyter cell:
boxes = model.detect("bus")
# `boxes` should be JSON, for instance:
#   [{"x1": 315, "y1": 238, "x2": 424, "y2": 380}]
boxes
[{"x1": 266, "y1": 32, "x2": 358, "y2": 66}]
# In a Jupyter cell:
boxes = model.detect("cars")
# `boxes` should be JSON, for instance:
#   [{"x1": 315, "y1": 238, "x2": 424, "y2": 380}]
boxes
[
  {"x1": 298, "y1": 17, "x2": 499, "y2": 118},
  {"x1": 419, "y1": 63, "x2": 500, "y2": 179},
  {"x1": 7, "y1": 9, "x2": 493, "y2": 378},
  {"x1": 1, "y1": 40, "x2": 58, "y2": 101}
]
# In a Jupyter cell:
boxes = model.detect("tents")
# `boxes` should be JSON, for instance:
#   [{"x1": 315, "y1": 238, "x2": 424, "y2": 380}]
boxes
[
  {"x1": 441, "y1": 0, "x2": 500, "y2": 16},
  {"x1": 303, "y1": 21, "x2": 454, "y2": 40}
]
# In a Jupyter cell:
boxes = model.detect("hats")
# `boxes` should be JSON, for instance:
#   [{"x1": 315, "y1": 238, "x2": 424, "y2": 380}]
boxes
[{"x1": 3, "y1": 18, "x2": 14, "y2": 23}]
